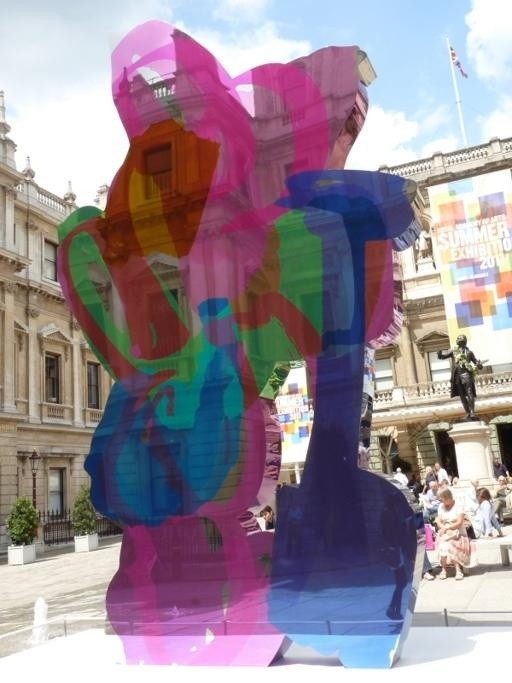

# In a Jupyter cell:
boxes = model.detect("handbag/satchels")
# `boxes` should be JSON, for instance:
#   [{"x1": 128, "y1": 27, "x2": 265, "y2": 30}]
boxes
[{"x1": 440, "y1": 520, "x2": 459, "y2": 541}]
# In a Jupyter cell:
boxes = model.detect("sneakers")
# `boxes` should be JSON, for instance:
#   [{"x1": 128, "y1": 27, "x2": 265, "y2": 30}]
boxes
[{"x1": 422, "y1": 568, "x2": 465, "y2": 580}]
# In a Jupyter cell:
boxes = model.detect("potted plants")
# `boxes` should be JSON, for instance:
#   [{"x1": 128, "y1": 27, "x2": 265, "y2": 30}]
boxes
[
  {"x1": 7, "y1": 497, "x2": 36, "y2": 565},
  {"x1": 70, "y1": 484, "x2": 98, "y2": 552}
]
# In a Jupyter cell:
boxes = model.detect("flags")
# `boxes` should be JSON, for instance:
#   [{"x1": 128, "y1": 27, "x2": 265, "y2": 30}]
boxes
[{"x1": 450, "y1": 46, "x2": 468, "y2": 78}]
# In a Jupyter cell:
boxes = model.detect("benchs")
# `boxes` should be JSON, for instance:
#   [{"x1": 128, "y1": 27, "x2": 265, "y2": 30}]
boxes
[{"x1": 470, "y1": 536, "x2": 511, "y2": 566}]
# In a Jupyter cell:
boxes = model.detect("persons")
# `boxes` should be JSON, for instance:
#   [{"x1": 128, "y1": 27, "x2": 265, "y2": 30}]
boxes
[
  {"x1": 260, "y1": 506, "x2": 276, "y2": 530},
  {"x1": 438, "y1": 335, "x2": 483, "y2": 420},
  {"x1": 394, "y1": 458, "x2": 510, "y2": 580}
]
